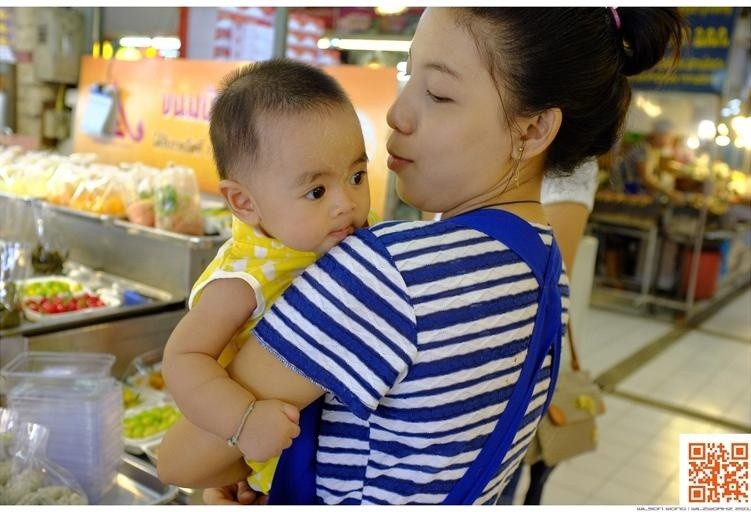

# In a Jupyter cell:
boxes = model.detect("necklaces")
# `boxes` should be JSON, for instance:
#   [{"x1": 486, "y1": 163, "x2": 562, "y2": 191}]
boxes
[{"x1": 439, "y1": 196, "x2": 546, "y2": 215}]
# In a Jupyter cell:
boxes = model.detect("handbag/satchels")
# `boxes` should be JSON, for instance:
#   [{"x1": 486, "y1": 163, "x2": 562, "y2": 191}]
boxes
[{"x1": 519, "y1": 363, "x2": 607, "y2": 467}]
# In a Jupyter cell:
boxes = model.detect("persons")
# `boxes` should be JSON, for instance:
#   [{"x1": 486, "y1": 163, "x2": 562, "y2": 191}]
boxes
[
  {"x1": 160, "y1": 61, "x2": 372, "y2": 460},
  {"x1": 600, "y1": 118, "x2": 686, "y2": 290},
  {"x1": 157, "y1": 7, "x2": 690, "y2": 504},
  {"x1": 538, "y1": 155, "x2": 601, "y2": 280}
]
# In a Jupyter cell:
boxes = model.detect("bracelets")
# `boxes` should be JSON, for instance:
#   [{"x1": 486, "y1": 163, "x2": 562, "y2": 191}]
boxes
[{"x1": 225, "y1": 395, "x2": 256, "y2": 447}]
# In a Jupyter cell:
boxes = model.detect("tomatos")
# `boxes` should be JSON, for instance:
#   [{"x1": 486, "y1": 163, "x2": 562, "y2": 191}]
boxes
[{"x1": 22, "y1": 291, "x2": 107, "y2": 314}]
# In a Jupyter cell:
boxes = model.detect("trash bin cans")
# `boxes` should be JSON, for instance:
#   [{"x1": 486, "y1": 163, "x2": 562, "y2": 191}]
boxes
[{"x1": 676, "y1": 238, "x2": 724, "y2": 300}]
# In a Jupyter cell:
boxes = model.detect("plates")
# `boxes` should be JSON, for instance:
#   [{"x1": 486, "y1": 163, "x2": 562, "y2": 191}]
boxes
[
  {"x1": 112, "y1": 381, "x2": 182, "y2": 466},
  {"x1": 3, "y1": 274, "x2": 125, "y2": 322}
]
